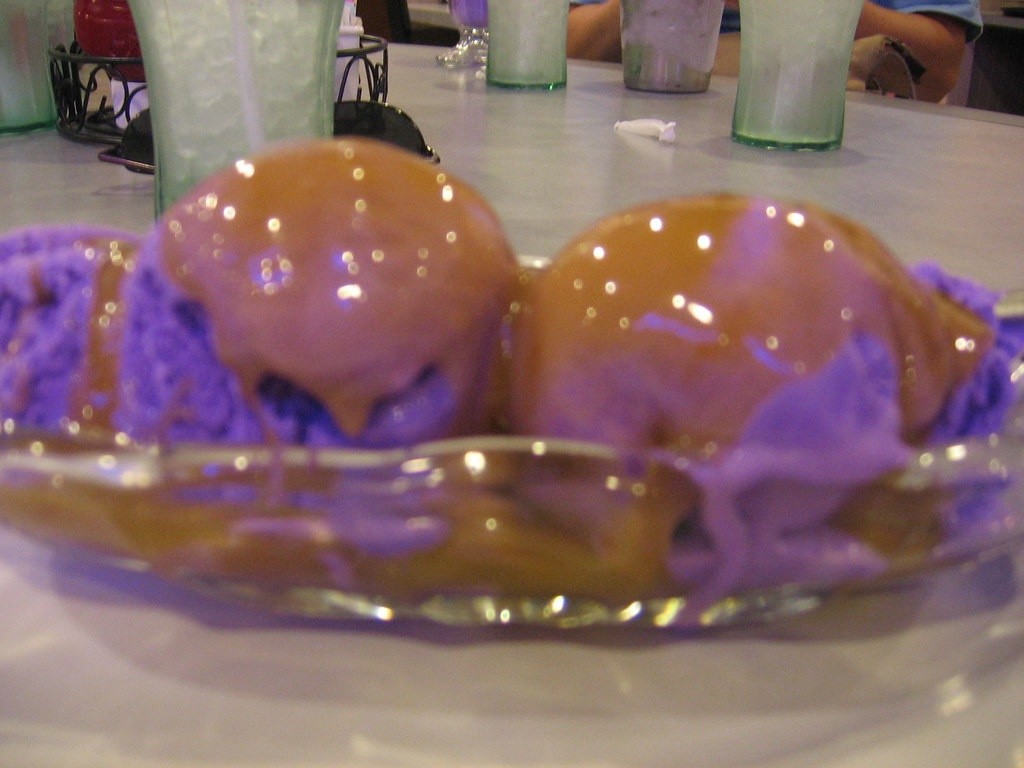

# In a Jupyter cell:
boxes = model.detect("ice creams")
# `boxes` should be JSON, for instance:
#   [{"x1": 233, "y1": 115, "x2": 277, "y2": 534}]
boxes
[{"x1": 0, "y1": 142, "x2": 1024, "y2": 610}]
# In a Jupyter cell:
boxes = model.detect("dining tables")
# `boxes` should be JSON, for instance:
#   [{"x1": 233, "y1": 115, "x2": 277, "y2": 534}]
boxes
[{"x1": 0, "y1": 43, "x2": 1024, "y2": 768}]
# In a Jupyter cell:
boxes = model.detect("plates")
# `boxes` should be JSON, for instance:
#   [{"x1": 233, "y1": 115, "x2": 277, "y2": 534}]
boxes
[{"x1": 0, "y1": 257, "x2": 1024, "y2": 625}]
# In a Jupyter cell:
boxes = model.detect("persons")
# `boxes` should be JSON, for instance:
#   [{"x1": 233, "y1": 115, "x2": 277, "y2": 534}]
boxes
[{"x1": 566, "y1": 0, "x2": 983, "y2": 102}]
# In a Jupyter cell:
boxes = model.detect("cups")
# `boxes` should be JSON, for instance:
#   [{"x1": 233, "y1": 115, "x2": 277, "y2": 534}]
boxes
[
  {"x1": 618, "y1": 0, "x2": 724, "y2": 92},
  {"x1": 731, "y1": 0, "x2": 864, "y2": 152},
  {"x1": 485, "y1": 0, "x2": 569, "y2": 89},
  {"x1": 0, "y1": 0, "x2": 344, "y2": 229}
]
